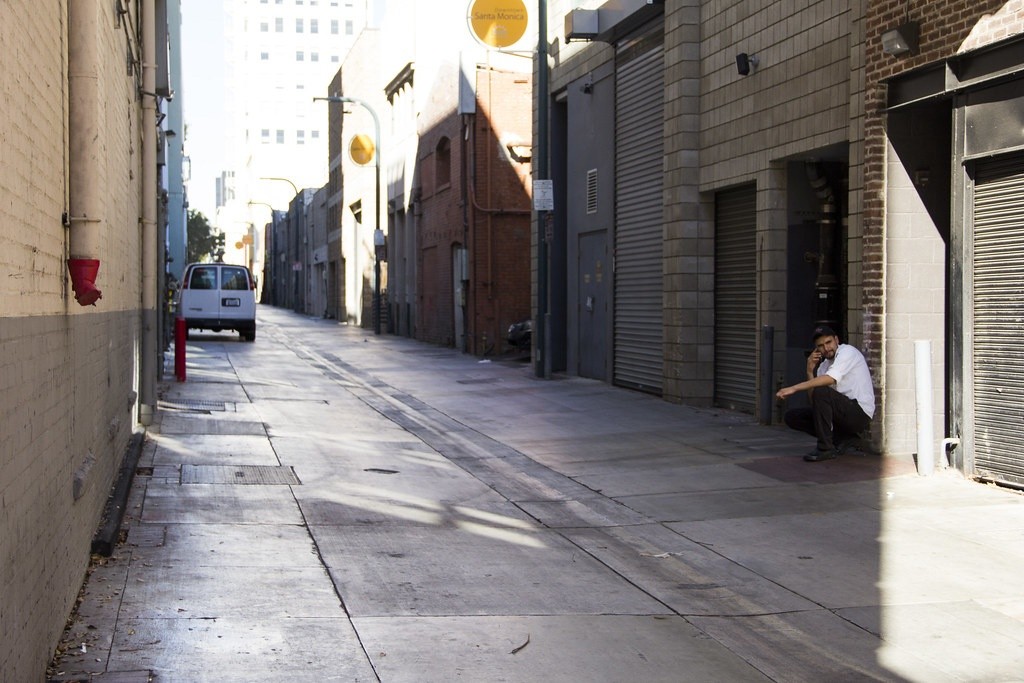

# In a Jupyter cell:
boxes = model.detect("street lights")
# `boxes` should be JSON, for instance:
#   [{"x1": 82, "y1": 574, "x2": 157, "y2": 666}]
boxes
[
  {"x1": 311, "y1": 95, "x2": 382, "y2": 334},
  {"x1": 258, "y1": 177, "x2": 300, "y2": 313},
  {"x1": 247, "y1": 201, "x2": 276, "y2": 307}
]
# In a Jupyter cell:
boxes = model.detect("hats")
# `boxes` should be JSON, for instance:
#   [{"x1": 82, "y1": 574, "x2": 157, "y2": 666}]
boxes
[{"x1": 813, "y1": 326, "x2": 834, "y2": 340}]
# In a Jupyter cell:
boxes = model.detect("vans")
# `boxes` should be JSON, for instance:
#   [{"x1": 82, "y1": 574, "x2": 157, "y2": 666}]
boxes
[{"x1": 175, "y1": 261, "x2": 258, "y2": 342}]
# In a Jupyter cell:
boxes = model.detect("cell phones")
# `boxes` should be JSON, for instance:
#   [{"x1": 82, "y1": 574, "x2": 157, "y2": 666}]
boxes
[{"x1": 816, "y1": 350, "x2": 822, "y2": 362}]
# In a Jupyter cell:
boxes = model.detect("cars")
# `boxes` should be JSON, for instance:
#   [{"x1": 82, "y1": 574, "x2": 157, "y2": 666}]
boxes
[{"x1": 509, "y1": 318, "x2": 532, "y2": 353}]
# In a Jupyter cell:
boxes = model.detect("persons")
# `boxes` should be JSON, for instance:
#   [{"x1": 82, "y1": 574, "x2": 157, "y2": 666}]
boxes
[
  {"x1": 167, "y1": 273, "x2": 180, "y2": 338},
  {"x1": 775, "y1": 326, "x2": 876, "y2": 463}
]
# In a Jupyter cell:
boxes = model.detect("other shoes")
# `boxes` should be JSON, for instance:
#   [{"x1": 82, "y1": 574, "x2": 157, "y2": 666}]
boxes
[
  {"x1": 803, "y1": 446, "x2": 836, "y2": 461},
  {"x1": 835, "y1": 434, "x2": 862, "y2": 454}
]
